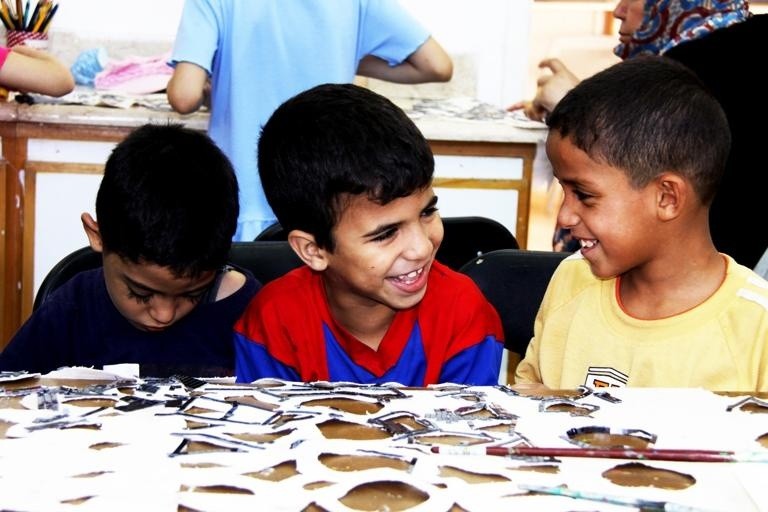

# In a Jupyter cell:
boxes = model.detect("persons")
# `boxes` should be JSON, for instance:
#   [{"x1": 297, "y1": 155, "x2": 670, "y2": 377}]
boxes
[
  {"x1": 507, "y1": 52, "x2": 768, "y2": 397},
  {"x1": 165, "y1": 0, "x2": 456, "y2": 257},
  {"x1": 522, "y1": 0, "x2": 768, "y2": 270},
  {"x1": 229, "y1": 82, "x2": 508, "y2": 387},
  {"x1": 2, "y1": 121, "x2": 265, "y2": 385},
  {"x1": 0, "y1": 43, "x2": 77, "y2": 99}
]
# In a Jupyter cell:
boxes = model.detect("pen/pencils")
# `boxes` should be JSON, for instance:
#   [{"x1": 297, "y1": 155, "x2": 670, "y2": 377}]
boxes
[
  {"x1": 486, "y1": 446, "x2": 735, "y2": 461},
  {"x1": 0, "y1": 0, "x2": 58, "y2": 32}
]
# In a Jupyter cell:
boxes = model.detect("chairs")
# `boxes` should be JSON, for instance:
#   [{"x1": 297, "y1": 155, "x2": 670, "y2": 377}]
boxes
[
  {"x1": 33, "y1": 241, "x2": 304, "y2": 315},
  {"x1": 457, "y1": 248, "x2": 573, "y2": 360},
  {"x1": 252, "y1": 217, "x2": 520, "y2": 272}
]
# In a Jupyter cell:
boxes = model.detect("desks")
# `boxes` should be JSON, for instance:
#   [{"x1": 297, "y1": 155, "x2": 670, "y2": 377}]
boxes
[
  {"x1": 1, "y1": 370, "x2": 768, "y2": 510},
  {"x1": 2, "y1": 82, "x2": 547, "y2": 346}
]
